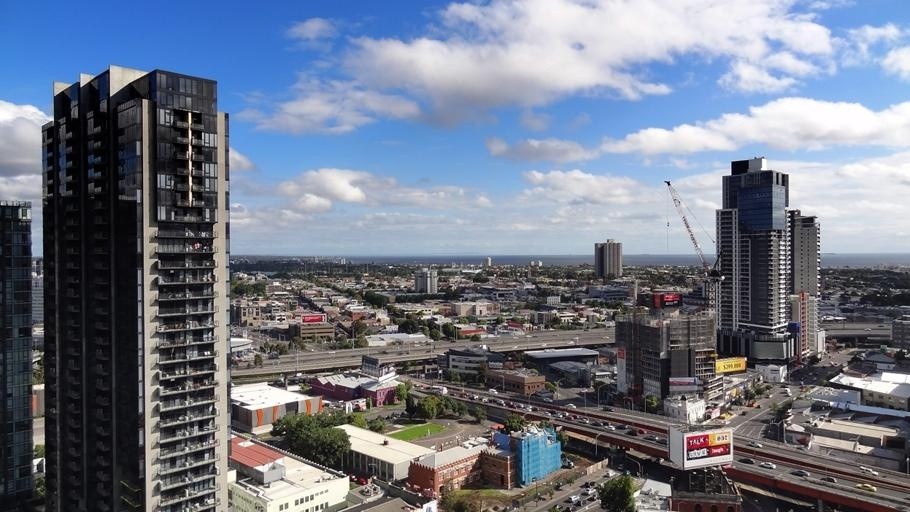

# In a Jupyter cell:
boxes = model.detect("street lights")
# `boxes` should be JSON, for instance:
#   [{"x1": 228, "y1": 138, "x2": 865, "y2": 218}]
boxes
[
  {"x1": 595, "y1": 431, "x2": 604, "y2": 456},
  {"x1": 597, "y1": 383, "x2": 611, "y2": 407}
]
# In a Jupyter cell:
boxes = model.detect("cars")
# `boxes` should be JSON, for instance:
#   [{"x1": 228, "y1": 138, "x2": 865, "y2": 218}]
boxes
[
  {"x1": 386, "y1": 328, "x2": 611, "y2": 355},
  {"x1": 349, "y1": 474, "x2": 367, "y2": 486},
  {"x1": 553, "y1": 464, "x2": 644, "y2": 512},
  {"x1": 738, "y1": 442, "x2": 878, "y2": 491},
  {"x1": 416, "y1": 383, "x2": 669, "y2": 446},
  {"x1": 740, "y1": 362, "x2": 839, "y2": 417}
]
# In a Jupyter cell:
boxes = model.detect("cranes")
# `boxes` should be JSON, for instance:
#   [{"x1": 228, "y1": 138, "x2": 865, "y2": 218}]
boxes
[{"x1": 661, "y1": 177, "x2": 723, "y2": 276}]
